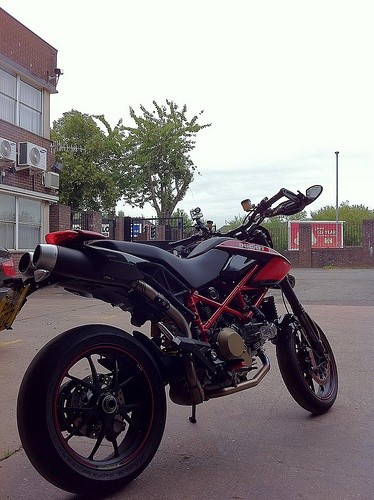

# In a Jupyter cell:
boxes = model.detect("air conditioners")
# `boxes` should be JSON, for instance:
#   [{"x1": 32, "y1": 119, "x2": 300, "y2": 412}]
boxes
[
  {"x1": 45, "y1": 171, "x2": 60, "y2": 190},
  {"x1": 18, "y1": 141, "x2": 47, "y2": 171},
  {"x1": 0, "y1": 137, "x2": 16, "y2": 162}
]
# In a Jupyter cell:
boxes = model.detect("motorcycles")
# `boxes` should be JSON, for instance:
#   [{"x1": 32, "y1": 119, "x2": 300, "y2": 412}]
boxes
[{"x1": 0, "y1": 183, "x2": 340, "y2": 500}]
